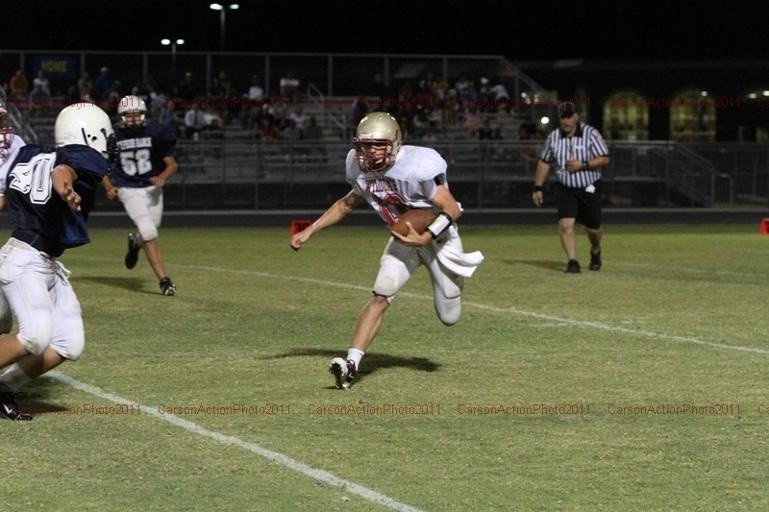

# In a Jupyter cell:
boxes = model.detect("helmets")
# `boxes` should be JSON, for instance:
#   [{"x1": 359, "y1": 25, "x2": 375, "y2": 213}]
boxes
[
  {"x1": 353, "y1": 112, "x2": 402, "y2": 174},
  {"x1": 54, "y1": 102, "x2": 120, "y2": 173},
  {"x1": 117, "y1": 94, "x2": 146, "y2": 129},
  {"x1": 0, "y1": 104, "x2": 16, "y2": 161}
]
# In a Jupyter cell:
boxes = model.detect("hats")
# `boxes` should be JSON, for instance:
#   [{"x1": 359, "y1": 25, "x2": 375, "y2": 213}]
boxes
[{"x1": 557, "y1": 101, "x2": 577, "y2": 118}]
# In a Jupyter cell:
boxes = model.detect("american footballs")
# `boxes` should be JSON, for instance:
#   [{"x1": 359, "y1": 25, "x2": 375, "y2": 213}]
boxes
[{"x1": 391, "y1": 210, "x2": 435, "y2": 237}]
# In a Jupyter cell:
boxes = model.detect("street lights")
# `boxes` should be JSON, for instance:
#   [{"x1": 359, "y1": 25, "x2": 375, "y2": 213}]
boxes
[
  {"x1": 209, "y1": 2, "x2": 240, "y2": 49},
  {"x1": 161, "y1": 37, "x2": 186, "y2": 93}
]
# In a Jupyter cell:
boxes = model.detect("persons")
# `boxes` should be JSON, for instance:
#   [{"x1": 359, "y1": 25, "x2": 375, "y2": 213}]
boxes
[
  {"x1": 0, "y1": 65, "x2": 547, "y2": 180},
  {"x1": 0, "y1": 101, "x2": 115, "y2": 421},
  {"x1": 530, "y1": 102, "x2": 609, "y2": 275},
  {"x1": 289, "y1": 114, "x2": 484, "y2": 390},
  {"x1": 100, "y1": 95, "x2": 179, "y2": 296}
]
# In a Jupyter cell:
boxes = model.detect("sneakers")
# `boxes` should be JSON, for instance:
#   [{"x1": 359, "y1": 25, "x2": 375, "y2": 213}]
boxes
[
  {"x1": 564, "y1": 259, "x2": 580, "y2": 273},
  {"x1": 159, "y1": 276, "x2": 175, "y2": 296},
  {"x1": 329, "y1": 358, "x2": 357, "y2": 390},
  {"x1": 125, "y1": 233, "x2": 139, "y2": 269},
  {"x1": 589, "y1": 245, "x2": 601, "y2": 271},
  {"x1": 0, "y1": 382, "x2": 33, "y2": 420}
]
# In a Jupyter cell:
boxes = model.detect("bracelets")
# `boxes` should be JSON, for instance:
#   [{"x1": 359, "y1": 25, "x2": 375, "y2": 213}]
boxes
[
  {"x1": 580, "y1": 160, "x2": 587, "y2": 170},
  {"x1": 532, "y1": 184, "x2": 544, "y2": 192},
  {"x1": 424, "y1": 211, "x2": 453, "y2": 240}
]
